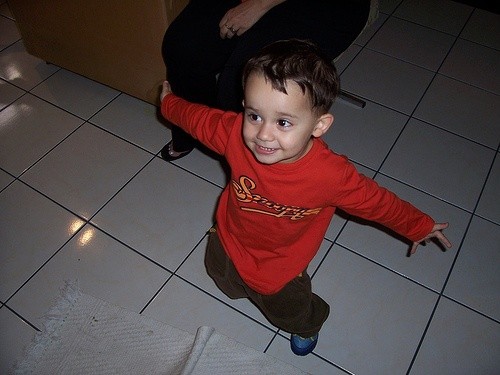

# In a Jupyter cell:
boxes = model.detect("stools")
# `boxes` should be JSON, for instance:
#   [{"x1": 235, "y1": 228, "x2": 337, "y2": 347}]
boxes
[{"x1": 325, "y1": 8, "x2": 370, "y2": 108}]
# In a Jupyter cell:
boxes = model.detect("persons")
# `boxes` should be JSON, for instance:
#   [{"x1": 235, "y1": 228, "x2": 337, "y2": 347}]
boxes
[
  {"x1": 161, "y1": 39, "x2": 452, "y2": 357},
  {"x1": 161, "y1": 0, "x2": 371, "y2": 161}
]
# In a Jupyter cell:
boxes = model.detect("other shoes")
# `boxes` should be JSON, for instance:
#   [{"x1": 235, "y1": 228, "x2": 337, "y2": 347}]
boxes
[{"x1": 161, "y1": 138, "x2": 195, "y2": 161}]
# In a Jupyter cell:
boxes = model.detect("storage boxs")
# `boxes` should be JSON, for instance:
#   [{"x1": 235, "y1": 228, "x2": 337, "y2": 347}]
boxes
[{"x1": 7, "y1": 0, "x2": 191, "y2": 110}]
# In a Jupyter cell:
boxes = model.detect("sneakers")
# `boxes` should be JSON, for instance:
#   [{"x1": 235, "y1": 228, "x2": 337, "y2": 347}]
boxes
[{"x1": 290, "y1": 332, "x2": 319, "y2": 358}]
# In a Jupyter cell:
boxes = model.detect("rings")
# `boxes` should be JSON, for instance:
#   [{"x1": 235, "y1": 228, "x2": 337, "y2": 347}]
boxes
[
  {"x1": 225, "y1": 24, "x2": 230, "y2": 29},
  {"x1": 229, "y1": 27, "x2": 236, "y2": 34}
]
